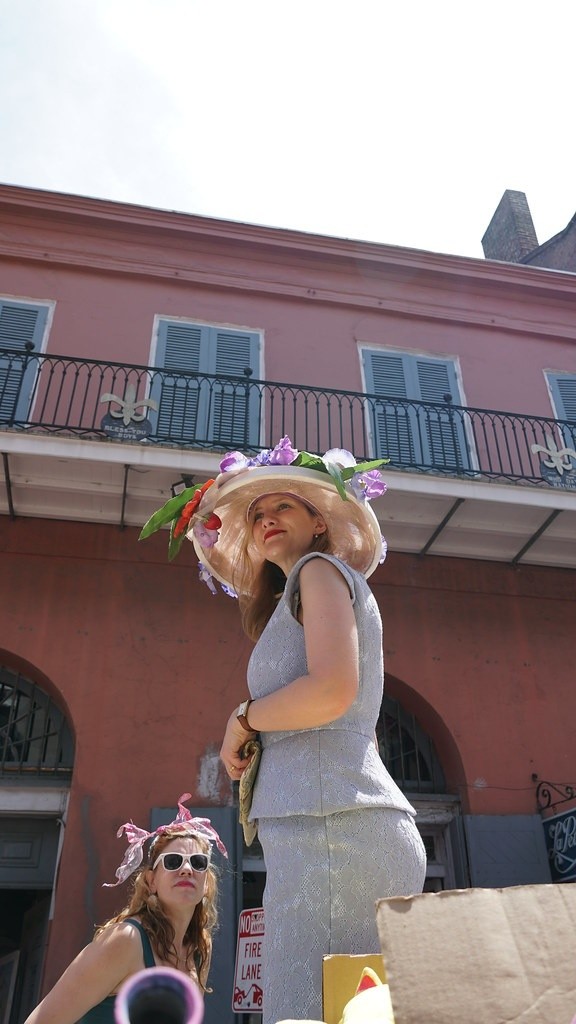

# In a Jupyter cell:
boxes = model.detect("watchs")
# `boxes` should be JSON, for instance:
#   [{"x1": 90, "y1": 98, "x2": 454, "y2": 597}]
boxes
[{"x1": 237, "y1": 699, "x2": 255, "y2": 733}]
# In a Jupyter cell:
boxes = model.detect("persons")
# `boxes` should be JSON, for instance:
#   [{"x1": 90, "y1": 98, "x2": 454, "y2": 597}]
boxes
[
  {"x1": 136, "y1": 436, "x2": 426, "y2": 1024},
  {"x1": 25, "y1": 793, "x2": 230, "y2": 1024}
]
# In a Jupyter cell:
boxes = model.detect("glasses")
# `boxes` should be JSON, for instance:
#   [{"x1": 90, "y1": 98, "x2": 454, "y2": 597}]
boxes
[{"x1": 153, "y1": 851, "x2": 210, "y2": 873}]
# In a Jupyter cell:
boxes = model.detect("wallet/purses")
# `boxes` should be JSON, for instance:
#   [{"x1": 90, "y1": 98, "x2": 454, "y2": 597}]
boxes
[{"x1": 239, "y1": 741, "x2": 261, "y2": 847}]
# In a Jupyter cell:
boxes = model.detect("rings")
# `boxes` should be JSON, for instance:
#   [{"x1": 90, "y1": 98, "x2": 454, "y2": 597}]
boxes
[{"x1": 230, "y1": 766, "x2": 235, "y2": 773}]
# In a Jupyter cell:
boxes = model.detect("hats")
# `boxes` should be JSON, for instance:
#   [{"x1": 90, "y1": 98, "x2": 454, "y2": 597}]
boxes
[{"x1": 138, "y1": 434, "x2": 388, "y2": 598}]
